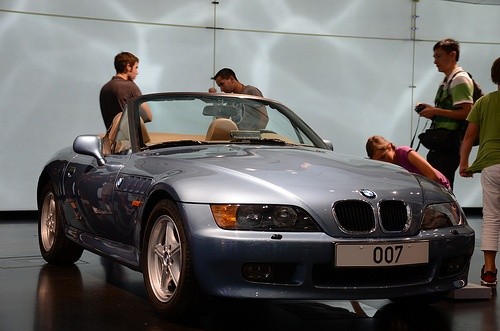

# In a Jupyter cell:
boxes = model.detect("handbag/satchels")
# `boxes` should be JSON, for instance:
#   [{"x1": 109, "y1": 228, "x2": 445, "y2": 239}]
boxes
[{"x1": 418, "y1": 128, "x2": 459, "y2": 151}]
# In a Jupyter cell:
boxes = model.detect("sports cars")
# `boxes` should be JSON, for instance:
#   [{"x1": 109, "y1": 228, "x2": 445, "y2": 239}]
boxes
[{"x1": 36, "y1": 92, "x2": 475, "y2": 319}]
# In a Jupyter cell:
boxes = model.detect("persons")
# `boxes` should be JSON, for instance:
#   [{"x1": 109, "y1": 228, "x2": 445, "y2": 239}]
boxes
[
  {"x1": 417, "y1": 39, "x2": 474, "y2": 191},
  {"x1": 366, "y1": 136, "x2": 454, "y2": 195},
  {"x1": 99, "y1": 52, "x2": 152, "y2": 132},
  {"x1": 460, "y1": 57, "x2": 500, "y2": 286},
  {"x1": 208, "y1": 68, "x2": 268, "y2": 131}
]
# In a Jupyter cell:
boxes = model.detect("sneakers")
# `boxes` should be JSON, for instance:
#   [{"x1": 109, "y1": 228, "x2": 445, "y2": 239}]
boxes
[{"x1": 480, "y1": 264, "x2": 498, "y2": 286}]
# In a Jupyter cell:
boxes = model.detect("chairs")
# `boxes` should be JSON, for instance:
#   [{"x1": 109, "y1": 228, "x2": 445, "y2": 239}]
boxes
[
  {"x1": 109, "y1": 112, "x2": 149, "y2": 147},
  {"x1": 205, "y1": 118, "x2": 239, "y2": 141}
]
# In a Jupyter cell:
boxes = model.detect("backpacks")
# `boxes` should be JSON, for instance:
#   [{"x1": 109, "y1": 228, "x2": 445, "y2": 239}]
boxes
[{"x1": 448, "y1": 72, "x2": 484, "y2": 145}]
